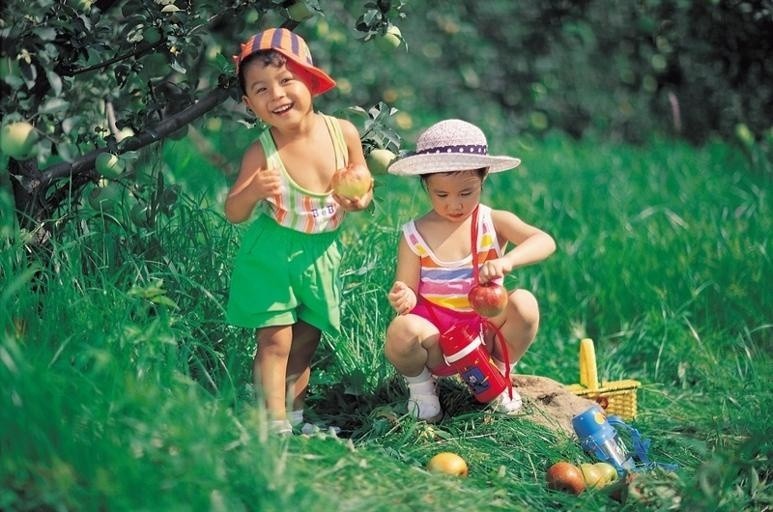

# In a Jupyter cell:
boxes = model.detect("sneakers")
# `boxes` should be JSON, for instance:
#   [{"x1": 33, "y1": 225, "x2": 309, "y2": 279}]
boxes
[
  {"x1": 406, "y1": 377, "x2": 442, "y2": 419},
  {"x1": 490, "y1": 386, "x2": 524, "y2": 415}
]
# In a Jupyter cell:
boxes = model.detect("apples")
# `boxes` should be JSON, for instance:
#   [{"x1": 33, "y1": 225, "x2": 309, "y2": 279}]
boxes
[
  {"x1": 367, "y1": 149, "x2": 397, "y2": 176},
  {"x1": 331, "y1": 163, "x2": 371, "y2": 202},
  {"x1": 468, "y1": 281, "x2": 507, "y2": 317},
  {"x1": 131, "y1": 203, "x2": 151, "y2": 228},
  {"x1": 0, "y1": 121, "x2": 40, "y2": 161},
  {"x1": 547, "y1": 462, "x2": 640, "y2": 501},
  {"x1": 429, "y1": 452, "x2": 468, "y2": 477},
  {"x1": 88, "y1": 188, "x2": 116, "y2": 211},
  {"x1": 374, "y1": 26, "x2": 402, "y2": 52},
  {"x1": 95, "y1": 151, "x2": 125, "y2": 179}
]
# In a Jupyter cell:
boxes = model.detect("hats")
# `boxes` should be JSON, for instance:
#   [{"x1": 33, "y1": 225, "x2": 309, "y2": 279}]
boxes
[
  {"x1": 232, "y1": 27, "x2": 337, "y2": 96},
  {"x1": 385, "y1": 117, "x2": 522, "y2": 177}
]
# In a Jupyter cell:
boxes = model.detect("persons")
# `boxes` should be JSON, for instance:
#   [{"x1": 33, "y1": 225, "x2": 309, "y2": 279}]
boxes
[
  {"x1": 384, "y1": 118, "x2": 556, "y2": 424},
  {"x1": 225, "y1": 28, "x2": 374, "y2": 437}
]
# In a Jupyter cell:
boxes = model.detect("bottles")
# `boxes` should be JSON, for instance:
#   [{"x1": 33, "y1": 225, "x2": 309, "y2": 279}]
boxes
[
  {"x1": 440, "y1": 323, "x2": 506, "y2": 402},
  {"x1": 571, "y1": 406, "x2": 637, "y2": 482}
]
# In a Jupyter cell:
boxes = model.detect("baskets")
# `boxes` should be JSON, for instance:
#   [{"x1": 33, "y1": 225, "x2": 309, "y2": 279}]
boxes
[{"x1": 563, "y1": 337, "x2": 642, "y2": 422}]
